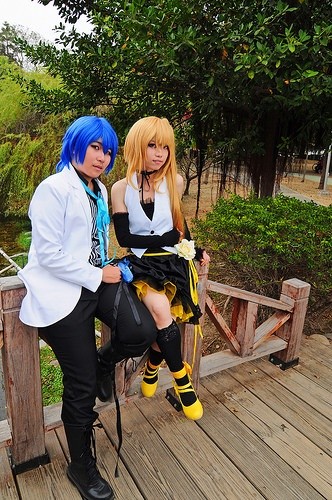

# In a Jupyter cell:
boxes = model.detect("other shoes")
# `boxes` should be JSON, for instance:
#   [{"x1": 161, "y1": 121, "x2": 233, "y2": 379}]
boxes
[
  {"x1": 170, "y1": 361, "x2": 204, "y2": 420},
  {"x1": 141, "y1": 359, "x2": 162, "y2": 398}
]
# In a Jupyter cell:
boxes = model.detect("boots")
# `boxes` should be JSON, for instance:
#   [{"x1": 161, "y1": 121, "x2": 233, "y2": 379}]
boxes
[
  {"x1": 64, "y1": 423, "x2": 114, "y2": 500},
  {"x1": 97, "y1": 341, "x2": 124, "y2": 402}
]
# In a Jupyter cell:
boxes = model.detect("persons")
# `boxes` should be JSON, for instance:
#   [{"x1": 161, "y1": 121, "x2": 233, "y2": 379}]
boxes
[
  {"x1": 16, "y1": 114, "x2": 158, "y2": 500},
  {"x1": 110, "y1": 115, "x2": 210, "y2": 421}
]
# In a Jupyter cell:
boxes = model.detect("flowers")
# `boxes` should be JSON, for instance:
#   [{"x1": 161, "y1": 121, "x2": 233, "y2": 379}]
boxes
[{"x1": 174, "y1": 238, "x2": 196, "y2": 260}]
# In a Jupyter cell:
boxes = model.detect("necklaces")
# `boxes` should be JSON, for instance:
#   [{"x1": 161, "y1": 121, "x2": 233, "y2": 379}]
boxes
[{"x1": 137, "y1": 170, "x2": 159, "y2": 204}]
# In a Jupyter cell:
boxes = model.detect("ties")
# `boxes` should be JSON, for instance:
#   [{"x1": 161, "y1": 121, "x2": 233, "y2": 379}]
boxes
[{"x1": 81, "y1": 179, "x2": 117, "y2": 268}]
